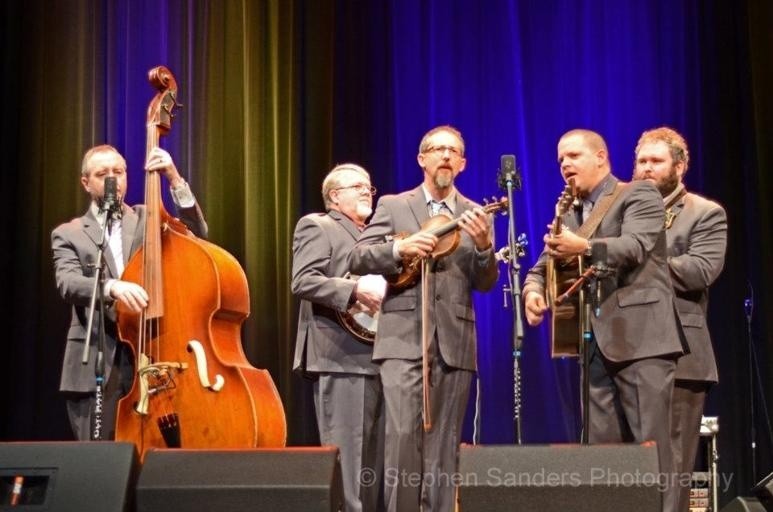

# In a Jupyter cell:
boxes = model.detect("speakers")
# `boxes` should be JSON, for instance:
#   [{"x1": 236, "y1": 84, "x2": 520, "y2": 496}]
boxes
[
  {"x1": 458, "y1": 442, "x2": 663, "y2": 512},
  {"x1": 0, "y1": 441, "x2": 142, "y2": 512},
  {"x1": 719, "y1": 496, "x2": 767, "y2": 512},
  {"x1": 135, "y1": 446, "x2": 346, "y2": 512}
]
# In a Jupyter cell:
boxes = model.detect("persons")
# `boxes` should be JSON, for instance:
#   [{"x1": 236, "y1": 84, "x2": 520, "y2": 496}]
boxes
[
  {"x1": 50, "y1": 143, "x2": 209, "y2": 439},
  {"x1": 631, "y1": 128, "x2": 729, "y2": 511},
  {"x1": 345, "y1": 125, "x2": 500, "y2": 510},
  {"x1": 290, "y1": 164, "x2": 387, "y2": 510},
  {"x1": 521, "y1": 129, "x2": 684, "y2": 510}
]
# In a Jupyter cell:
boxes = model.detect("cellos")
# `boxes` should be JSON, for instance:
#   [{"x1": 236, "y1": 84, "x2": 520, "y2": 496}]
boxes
[{"x1": 114, "y1": 66, "x2": 288, "y2": 465}]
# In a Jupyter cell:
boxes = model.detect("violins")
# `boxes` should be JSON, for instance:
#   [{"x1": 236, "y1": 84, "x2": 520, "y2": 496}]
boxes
[{"x1": 382, "y1": 196, "x2": 508, "y2": 287}]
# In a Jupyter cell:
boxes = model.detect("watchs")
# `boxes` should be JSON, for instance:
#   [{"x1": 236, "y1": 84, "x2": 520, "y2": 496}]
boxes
[{"x1": 585, "y1": 240, "x2": 593, "y2": 258}]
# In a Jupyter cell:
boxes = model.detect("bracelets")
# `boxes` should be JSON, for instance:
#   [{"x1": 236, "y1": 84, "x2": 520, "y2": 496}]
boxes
[
  {"x1": 171, "y1": 178, "x2": 186, "y2": 192},
  {"x1": 476, "y1": 242, "x2": 493, "y2": 252}
]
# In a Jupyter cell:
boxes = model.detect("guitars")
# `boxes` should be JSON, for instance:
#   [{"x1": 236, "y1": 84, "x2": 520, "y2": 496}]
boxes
[{"x1": 546, "y1": 179, "x2": 583, "y2": 358}]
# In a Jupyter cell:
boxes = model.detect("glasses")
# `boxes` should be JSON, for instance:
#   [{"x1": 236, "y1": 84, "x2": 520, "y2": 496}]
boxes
[{"x1": 336, "y1": 183, "x2": 377, "y2": 195}]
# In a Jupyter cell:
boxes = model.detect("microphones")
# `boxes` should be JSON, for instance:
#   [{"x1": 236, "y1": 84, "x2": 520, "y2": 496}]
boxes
[
  {"x1": 104, "y1": 177, "x2": 117, "y2": 211},
  {"x1": 500, "y1": 154, "x2": 516, "y2": 192},
  {"x1": 743, "y1": 282, "x2": 755, "y2": 325},
  {"x1": 592, "y1": 243, "x2": 606, "y2": 317}
]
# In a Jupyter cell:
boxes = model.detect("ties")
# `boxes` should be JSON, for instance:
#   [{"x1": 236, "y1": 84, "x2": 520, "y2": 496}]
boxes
[
  {"x1": 583, "y1": 200, "x2": 593, "y2": 223},
  {"x1": 430, "y1": 202, "x2": 442, "y2": 215}
]
